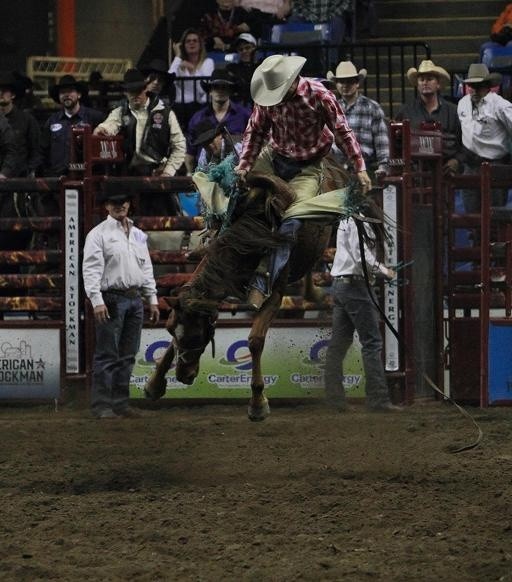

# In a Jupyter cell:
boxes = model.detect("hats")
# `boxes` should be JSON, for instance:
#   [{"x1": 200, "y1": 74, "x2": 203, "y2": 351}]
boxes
[
  {"x1": 454, "y1": 62, "x2": 503, "y2": 87},
  {"x1": 201, "y1": 69, "x2": 246, "y2": 95},
  {"x1": 234, "y1": 32, "x2": 257, "y2": 48},
  {"x1": 402, "y1": 58, "x2": 452, "y2": 88},
  {"x1": 98, "y1": 181, "x2": 136, "y2": 206},
  {"x1": 118, "y1": 69, "x2": 149, "y2": 90},
  {"x1": 140, "y1": 58, "x2": 177, "y2": 85},
  {"x1": 325, "y1": 59, "x2": 368, "y2": 86},
  {"x1": 48, "y1": 74, "x2": 89, "y2": 105},
  {"x1": 0, "y1": 70, "x2": 34, "y2": 91}
]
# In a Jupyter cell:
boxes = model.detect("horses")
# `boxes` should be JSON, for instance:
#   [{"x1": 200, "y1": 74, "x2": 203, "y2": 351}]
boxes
[{"x1": 143, "y1": 152, "x2": 410, "y2": 422}]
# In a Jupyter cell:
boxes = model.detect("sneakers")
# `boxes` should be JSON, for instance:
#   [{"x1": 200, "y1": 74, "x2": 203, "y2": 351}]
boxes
[
  {"x1": 90, "y1": 404, "x2": 139, "y2": 419},
  {"x1": 317, "y1": 399, "x2": 349, "y2": 413},
  {"x1": 368, "y1": 400, "x2": 406, "y2": 415},
  {"x1": 247, "y1": 286, "x2": 267, "y2": 312},
  {"x1": 186, "y1": 229, "x2": 217, "y2": 261}
]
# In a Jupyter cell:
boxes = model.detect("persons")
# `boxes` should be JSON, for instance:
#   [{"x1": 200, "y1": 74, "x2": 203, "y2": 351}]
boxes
[
  {"x1": 323, "y1": 212, "x2": 405, "y2": 412},
  {"x1": 82, "y1": 182, "x2": 161, "y2": 419}
]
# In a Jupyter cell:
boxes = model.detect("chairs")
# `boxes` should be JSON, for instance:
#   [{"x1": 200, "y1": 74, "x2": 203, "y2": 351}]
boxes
[
  {"x1": 479, "y1": 40, "x2": 512, "y2": 72},
  {"x1": 183, "y1": 0, "x2": 377, "y2": 63}
]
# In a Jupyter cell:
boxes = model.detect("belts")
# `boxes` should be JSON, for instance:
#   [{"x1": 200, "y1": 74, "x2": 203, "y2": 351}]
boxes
[
  {"x1": 106, "y1": 288, "x2": 143, "y2": 299},
  {"x1": 334, "y1": 274, "x2": 367, "y2": 283}
]
